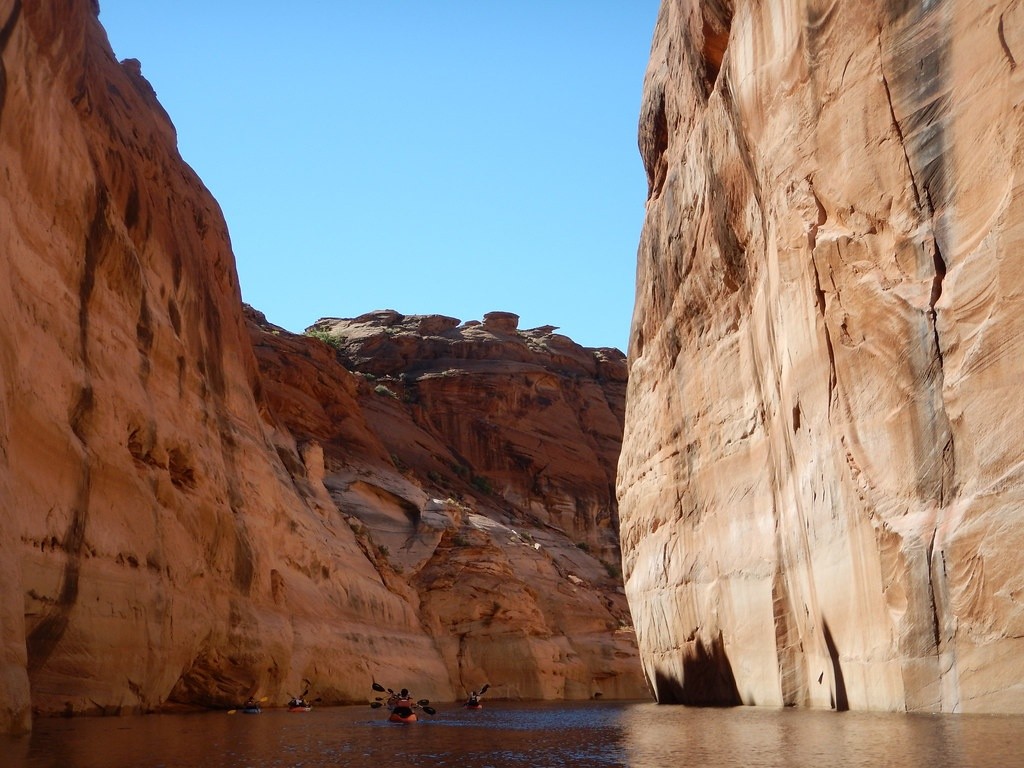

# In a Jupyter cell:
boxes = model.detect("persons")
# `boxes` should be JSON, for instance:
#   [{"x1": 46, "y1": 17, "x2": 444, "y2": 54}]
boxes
[
  {"x1": 247, "y1": 697, "x2": 254, "y2": 706},
  {"x1": 288, "y1": 698, "x2": 299, "y2": 708},
  {"x1": 296, "y1": 695, "x2": 309, "y2": 707},
  {"x1": 386, "y1": 689, "x2": 414, "y2": 711},
  {"x1": 469, "y1": 691, "x2": 477, "y2": 703}
]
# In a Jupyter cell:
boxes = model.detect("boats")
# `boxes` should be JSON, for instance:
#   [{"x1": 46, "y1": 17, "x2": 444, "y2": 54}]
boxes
[
  {"x1": 462, "y1": 700, "x2": 484, "y2": 711},
  {"x1": 388, "y1": 713, "x2": 419, "y2": 723},
  {"x1": 287, "y1": 704, "x2": 315, "y2": 716},
  {"x1": 244, "y1": 706, "x2": 264, "y2": 715}
]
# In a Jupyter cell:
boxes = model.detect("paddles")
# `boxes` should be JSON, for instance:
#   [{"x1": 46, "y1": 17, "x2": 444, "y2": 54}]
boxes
[
  {"x1": 302, "y1": 689, "x2": 309, "y2": 697},
  {"x1": 370, "y1": 683, "x2": 437, "y2": 716},
  {"x1": 459, "y1": 683, "x2": 491, "y2": 710},
  {"x1": 226, "y1": 696, "x2": 268, "y2": 716},
  {"x1": 309, "y1": 696, "x2": 323, "y2": 703}
]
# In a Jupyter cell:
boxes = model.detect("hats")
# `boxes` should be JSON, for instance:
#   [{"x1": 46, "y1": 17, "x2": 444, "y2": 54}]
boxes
[{"x1": 248, "y1": 696, "x2": 254, "y2": 700}]
